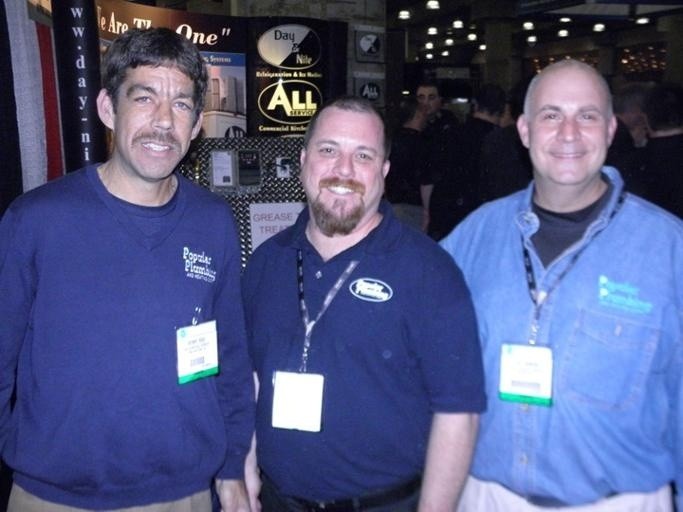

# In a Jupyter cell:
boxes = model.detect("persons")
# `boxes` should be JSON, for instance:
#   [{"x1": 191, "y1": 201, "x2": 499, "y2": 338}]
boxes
[
  {"x1": 440, "y1": 58, "x2": 683, "y2": 511},
  {"x1": 233, "y1": 93, "x2": 487, "y2": 512},
  {"x1": 382, "y1": 75, "x2": 532, "y2": 241},
  {"x1": 603, "y1": 73, "x2": 681, "y2": 219},
  {"x1": 0, "y1": 27, "x2": 258, "y2": 511}
]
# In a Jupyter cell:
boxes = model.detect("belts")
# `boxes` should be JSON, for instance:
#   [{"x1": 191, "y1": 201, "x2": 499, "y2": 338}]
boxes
[{"x1": 292, "y1": 477, "x2": 420, "y2": 512}]
